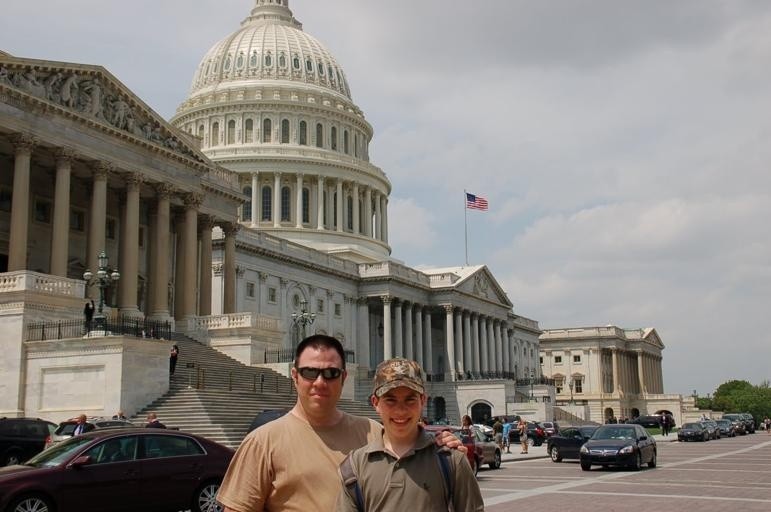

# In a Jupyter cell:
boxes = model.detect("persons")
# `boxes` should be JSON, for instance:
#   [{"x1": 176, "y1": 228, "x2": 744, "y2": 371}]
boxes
[
  {"x1": 71, "y1": 414, "x2": 95, "y2": 437},
  {"x1": 660, "y1": 411, "x2": 670, "y2": 436},
  {"x1": 215, "y1": 335, "x2": 468, "y2": 512},
  {"x1": 460, "y1": 415, "x2": 476, "y2": 468},
  {"x1": 502, "y1": 419, "x2": 511, "y2": 453},
  {"x1": 763, "y1": 416, "x2": 771, "y2": 433},
  {"x1": 517, "y1": 420, "x2": 528, "y2": 454},
  {"x1": 170, "y1": 345, "x2": 179, "y2": 376},
  {"x1": 608, "y1": 415, "x2": 638, "y2": 424},
  {"x1": 334, "y1": 356, "x2": 485, "y2": 512},
  {"x1": 112, "y1": 410, "x2": 126, "y2": 421},
  {"x1": 492, "y1": 417, "x2": 503, "y2": 448},
  {"x1": 83, "y1": 299, "x2": 94, "y2": 332},
  {"x1": 145, "y1": 412, "x2": 166, "y2": 428}
]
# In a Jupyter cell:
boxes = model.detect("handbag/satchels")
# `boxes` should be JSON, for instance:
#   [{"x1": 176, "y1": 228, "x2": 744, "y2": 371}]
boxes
[{"x1": 518, "y1": 430, "x2": 523, "y2": 436}]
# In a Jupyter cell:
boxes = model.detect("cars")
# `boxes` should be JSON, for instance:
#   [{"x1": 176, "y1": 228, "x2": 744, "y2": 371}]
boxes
[
  {"x1": 1, "y1": 425, "x2": 241, "y2": 512},
  {"x1": 675, "y1": 412, "x2": 756, "y2": 444},
  {"x1": 545, "y1": 422, "x2": 600, "y2": 465},
  {"x1": 423, "y1": 411, "x2": 556, "y2": 480},
  {"x1": 578, "y1": 423, "x2": 659, "y2": 472}
]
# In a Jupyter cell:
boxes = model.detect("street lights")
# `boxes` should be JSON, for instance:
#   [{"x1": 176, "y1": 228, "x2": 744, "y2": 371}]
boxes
[
  {"x1": 568, "y1": 380, "x2": 577, "y2": 406},
  {"x1": 289, "y1": 297, "x2": 318, "y2": 340},
  {"x1": 81, "y1": 248, "x2": 122, "y2": 338},
  {"x1": 529, "y1": 367, "x2": 534, "y2": 399}
]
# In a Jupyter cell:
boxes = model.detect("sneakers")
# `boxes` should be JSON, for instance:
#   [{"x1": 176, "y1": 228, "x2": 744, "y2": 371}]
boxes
[{"x1": 500, "y1": 448, "x2": 528, "y2": 454}]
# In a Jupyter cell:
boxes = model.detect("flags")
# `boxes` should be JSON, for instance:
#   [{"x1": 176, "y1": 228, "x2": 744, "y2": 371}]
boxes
[{"x1": 466, "y1": 192, "x2": 488, "y2": 212}]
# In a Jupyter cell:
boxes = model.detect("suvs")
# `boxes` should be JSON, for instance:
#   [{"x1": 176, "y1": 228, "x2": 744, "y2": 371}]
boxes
[
  {"x1": 0, "y1": 416, "x2": 61, "y2": 466},
  {"x1": 42, "y1": 414, "x2": 137, "y2": 454},
  {"x1": 627, "y1": 413, "x2": 676, "y2": 428}
]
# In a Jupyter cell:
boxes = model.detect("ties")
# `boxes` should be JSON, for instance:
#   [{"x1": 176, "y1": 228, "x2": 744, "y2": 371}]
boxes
[{"x1": 78, "y1": 426, "x2": 82, "y2": 434}]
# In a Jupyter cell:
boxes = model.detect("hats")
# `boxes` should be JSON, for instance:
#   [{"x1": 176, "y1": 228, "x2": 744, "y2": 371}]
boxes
[{"x1": 372, "y1": 356, "x2": 424, "y2": 397}]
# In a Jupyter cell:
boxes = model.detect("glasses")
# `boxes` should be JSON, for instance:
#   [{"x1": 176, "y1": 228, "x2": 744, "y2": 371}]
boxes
[{"x1": 295, "y1": 367, "x2": 344, "y2": 380}]
